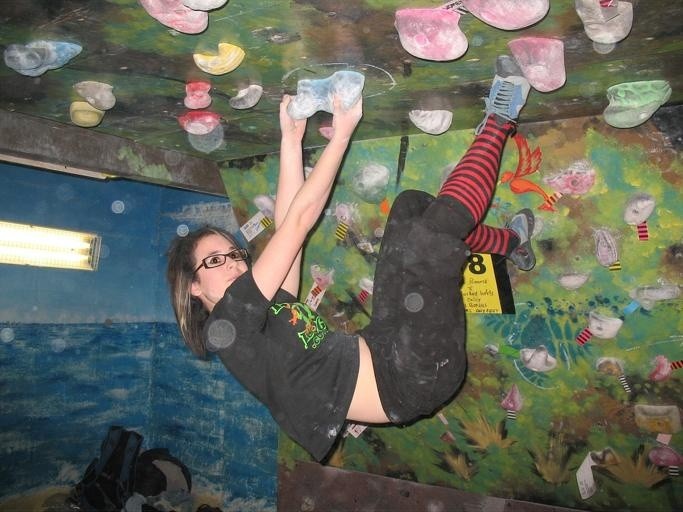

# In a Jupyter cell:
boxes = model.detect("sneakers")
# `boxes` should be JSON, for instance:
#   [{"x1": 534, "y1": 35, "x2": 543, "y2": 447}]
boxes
[
  {"x1": 505, "y1": 207, "x2": 537, "y2": 272},
  {"x1": 484, "y1": 52, "x2": 532, "y2": 127}
]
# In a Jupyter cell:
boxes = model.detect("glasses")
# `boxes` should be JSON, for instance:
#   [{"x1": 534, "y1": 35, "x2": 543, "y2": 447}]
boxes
[{"x1": 192, "y1": 248, "x2": 253, "y2": 277}]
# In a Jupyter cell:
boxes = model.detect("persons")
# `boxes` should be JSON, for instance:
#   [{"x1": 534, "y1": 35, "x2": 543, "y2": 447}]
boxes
[{"x1": 162, "y1": 76, "x2": 538, "y2": 464}]
